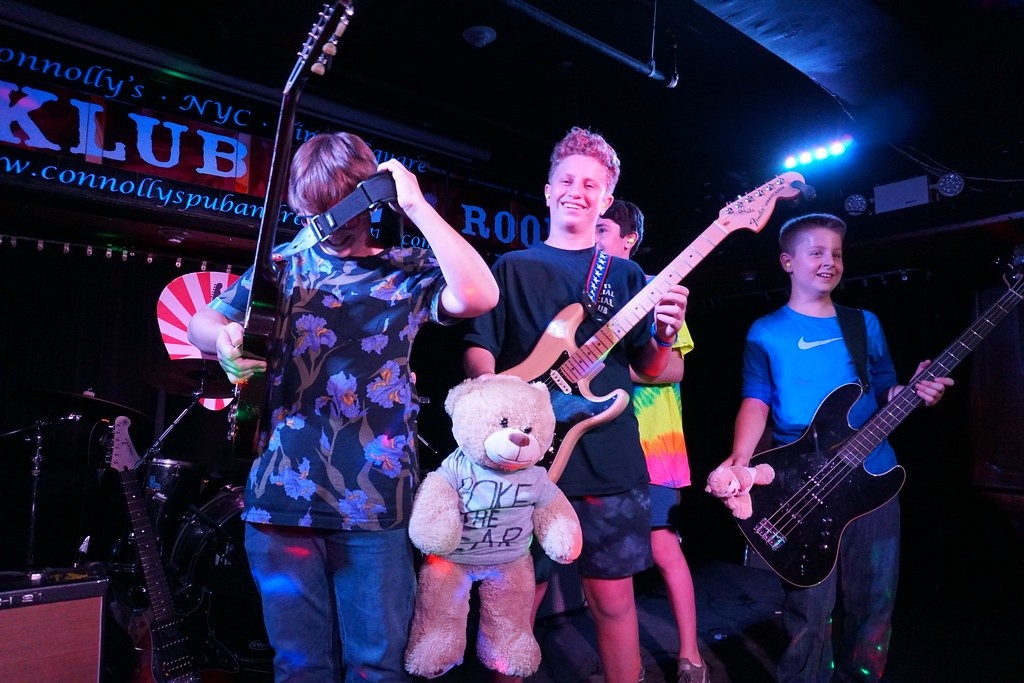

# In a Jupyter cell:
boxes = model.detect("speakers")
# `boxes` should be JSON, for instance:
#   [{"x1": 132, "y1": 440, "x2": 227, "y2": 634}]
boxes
[{"x1": 0, "y1": 571, "x2": 109, "y2": 683}]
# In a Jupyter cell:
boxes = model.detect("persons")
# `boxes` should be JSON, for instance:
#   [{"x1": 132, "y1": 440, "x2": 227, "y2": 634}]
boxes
[
  {"x1": 184, "y1": 131, "x2": 502, "y2": 683},
  {"x1": 455, "y1": 124, "x2": 710, "y2": 683},
  {"x1": 716, "y1": 212, "x2": 956, "y2": 683}
]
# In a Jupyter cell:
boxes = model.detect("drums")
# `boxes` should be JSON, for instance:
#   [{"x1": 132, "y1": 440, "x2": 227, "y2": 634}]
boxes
[
  {"x1": 161, "y1": 483, "x2": 278, "y2": 677},
  {"x1": 137, "y1": 457, "x2": 197, "y2": 524}
]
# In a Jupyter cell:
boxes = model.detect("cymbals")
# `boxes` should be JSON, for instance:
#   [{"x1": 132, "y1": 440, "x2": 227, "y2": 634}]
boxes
[{"x1": 47, "y1": 386, "x2": 150, "y2": 423}]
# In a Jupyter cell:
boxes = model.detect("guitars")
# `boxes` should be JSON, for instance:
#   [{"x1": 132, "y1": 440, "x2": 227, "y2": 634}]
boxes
[
  {"x1": 101, "y1": 414, "x2": 245, "y2": 683},
  {"x1": 222, "y1": 0, "x2": 357, "y2": 462},
  {"x1": 716, "y1": 262, "x2": 1024, "y2": 590},
  {"x1": 492, "y1": 167, "x2": 818, "y2": 486}
]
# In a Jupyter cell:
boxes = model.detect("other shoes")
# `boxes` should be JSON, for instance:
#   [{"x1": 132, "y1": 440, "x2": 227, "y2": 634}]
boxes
[
  {"x1": 678, "y1": 655, "x2": 709, "y2": 683},
  {"x1": 589, "y1": 656, "x2": 645, "y2": 683}
]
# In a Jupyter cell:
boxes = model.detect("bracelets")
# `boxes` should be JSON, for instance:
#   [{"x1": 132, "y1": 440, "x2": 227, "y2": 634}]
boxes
[{"x1": 649, "y1": 322, "x2": 679, "y2": 348}]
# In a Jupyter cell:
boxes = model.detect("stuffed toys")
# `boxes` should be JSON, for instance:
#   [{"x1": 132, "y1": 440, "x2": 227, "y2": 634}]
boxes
[
  {"x1": 402, "y1": 371, "x2": 584, "y2": 680},
  {"x1": 704, "y1": 462, "x2": 776, "y2": 522}
]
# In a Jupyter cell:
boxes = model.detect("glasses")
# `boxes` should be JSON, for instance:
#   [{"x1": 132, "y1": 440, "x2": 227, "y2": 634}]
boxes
[{"x1": 294, "y1": 209, "x2": 371, "y2": 230}]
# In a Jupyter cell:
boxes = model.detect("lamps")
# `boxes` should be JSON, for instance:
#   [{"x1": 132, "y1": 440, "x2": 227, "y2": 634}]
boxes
[{"x1": 844, "y1": 172, "x2": 965, "y2": 217}]
